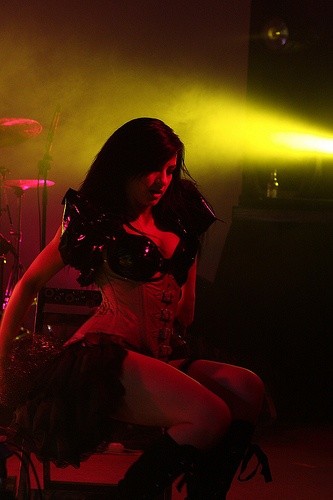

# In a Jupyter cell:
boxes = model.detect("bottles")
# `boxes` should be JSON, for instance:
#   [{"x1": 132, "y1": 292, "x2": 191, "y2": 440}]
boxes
[{"x1": 265, "y1": 172, "x2": 279, "y2": 199}]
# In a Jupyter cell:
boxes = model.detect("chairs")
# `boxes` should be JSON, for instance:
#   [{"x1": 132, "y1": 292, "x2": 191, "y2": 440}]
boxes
[{"x1": 0, "y1": 286, "x2": 172, "y2": 500}]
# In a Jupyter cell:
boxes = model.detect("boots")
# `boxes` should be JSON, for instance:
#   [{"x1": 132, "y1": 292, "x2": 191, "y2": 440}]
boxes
[{"x1": 112, "y1": 419, "x2": 257, "y2": 500}]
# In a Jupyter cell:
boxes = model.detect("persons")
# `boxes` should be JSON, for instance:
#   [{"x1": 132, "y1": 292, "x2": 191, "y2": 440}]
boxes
[{"x1": 1, "y1": 118, "x2": 266, "y2": 500}]
